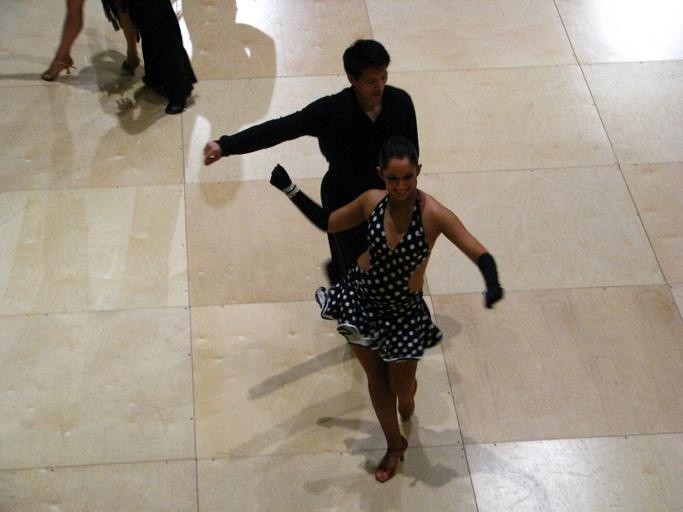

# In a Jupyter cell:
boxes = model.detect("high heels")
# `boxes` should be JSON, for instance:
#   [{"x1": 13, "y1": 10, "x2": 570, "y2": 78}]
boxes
[
  {"x1": 41, "y1": 60, "x2": 70, "y2": 81},
  {"x1": 375, "y1": 435, "x2": 407, "y2": 482},
  {"x1": 123, "y1": 57, "x2": 140, "y2": 74}
]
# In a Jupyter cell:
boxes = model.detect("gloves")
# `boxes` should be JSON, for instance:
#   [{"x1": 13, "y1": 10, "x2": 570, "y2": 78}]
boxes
[
  {"x1": 270, "y1": 163, "x2": 330, "y2": 231},
  {"x1": 477, "y1": 253, "x2": 502, "y2": 309}
]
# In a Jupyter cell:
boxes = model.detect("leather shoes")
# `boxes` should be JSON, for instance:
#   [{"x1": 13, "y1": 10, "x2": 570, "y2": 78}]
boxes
[{"x1": 165, "y1": 93, "x2": 187, "y2": 114}]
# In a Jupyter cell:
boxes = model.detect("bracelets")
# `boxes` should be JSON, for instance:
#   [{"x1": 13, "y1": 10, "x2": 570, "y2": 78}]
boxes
[
  {"x1": 288, "y1": 185, "x2": 300, "y2": 199},
  {"x1": 283, "y1": 183, "x2": 295, "y2": 192}
]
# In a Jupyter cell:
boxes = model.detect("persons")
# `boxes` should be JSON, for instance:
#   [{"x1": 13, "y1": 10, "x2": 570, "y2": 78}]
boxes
[
  {"x1": 41, "y1": 0, "x2": 140, "y2": 81},
  {"x1": 269, "y1": 136, "x2": 502, "y2": 482},
  {"x1": 204, "y1": 40, "x2": 419, "y2": 286},
  {"x1": 130, "y1": 0, "x2": 197, "y2": 114}
]
